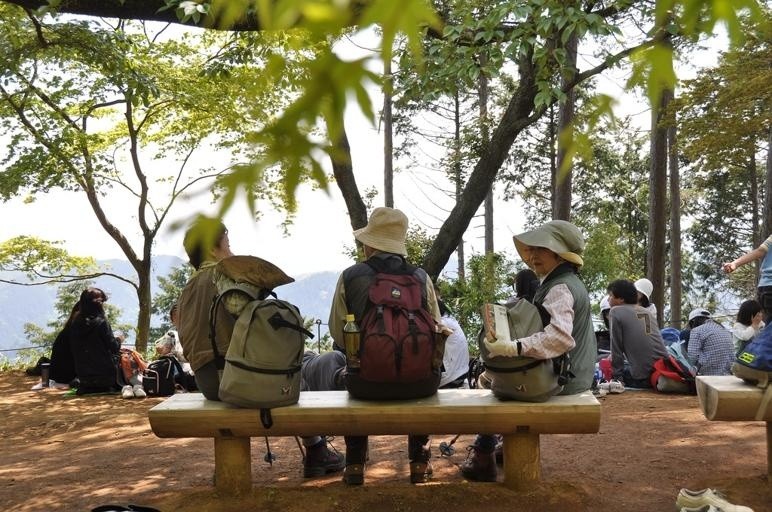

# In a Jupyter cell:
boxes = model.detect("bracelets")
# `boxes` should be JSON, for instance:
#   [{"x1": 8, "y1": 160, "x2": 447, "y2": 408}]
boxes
[{"x1": 516, "y1": 338, "x2": 522, "y2": 357}]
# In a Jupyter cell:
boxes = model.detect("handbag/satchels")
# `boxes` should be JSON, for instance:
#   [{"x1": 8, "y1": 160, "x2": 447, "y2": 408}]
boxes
[{"x1": 595, "y1": 331, "x2": 610, "y2": 354}]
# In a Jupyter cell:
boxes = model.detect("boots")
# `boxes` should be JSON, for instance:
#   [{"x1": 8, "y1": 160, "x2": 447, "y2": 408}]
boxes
[
  {"x1": 304, "y1": 439, "x2": 346, "y2": 478},
  {"x1": 459, "y1": 445, "x2": 497, "y2": 482}
]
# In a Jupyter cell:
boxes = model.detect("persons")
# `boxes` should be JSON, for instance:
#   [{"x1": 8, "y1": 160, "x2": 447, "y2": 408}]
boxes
[
  {"x1": 175, "y1": 215, "x2": 346, "y2": 480},
  {"x1": 327, "y1": 205, "x2": 442, "y2": 486},
  {"x1": 70, "y1": 287, "x2": 133, "y2": 395},
  {"x1": 461, "y1": 220, "x2": 599, "y2": 480},
  {"x1": 430, "y1": 291, "x2": 470, "y2": 389},
  {"x1": 300, "y1": 346, "x2": 349, "y2": 391},
  {"x1": 50, "y1": 300, "x2": 82, "y2": 388},
  {"x1": 600, "y1": 230, "x2": 772, "y2": 397},
  {"x1": 156, "y1": 303, "x2": 198, "y2": 392},
  {"x1": 513, "y1": 268, "x2": 539, "y2": 307}
]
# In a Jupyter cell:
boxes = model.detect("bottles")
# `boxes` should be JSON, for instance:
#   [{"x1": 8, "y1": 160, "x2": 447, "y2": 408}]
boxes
[
  {"x1": 343, "y1": 315, "x2": 361, "y2": 366},
  {"x1": 41, "y1": 364, "x2": 50, "y2": 387}
]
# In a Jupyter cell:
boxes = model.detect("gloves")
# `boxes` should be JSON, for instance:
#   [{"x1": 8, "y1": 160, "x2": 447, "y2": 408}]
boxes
[{"x1": 482, "y1": 336, "x2": 520, "y2": 359}]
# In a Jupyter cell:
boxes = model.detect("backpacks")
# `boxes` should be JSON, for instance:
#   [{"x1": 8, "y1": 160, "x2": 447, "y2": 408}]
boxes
[
  {"x1": 209, "y1": 284, "x2": 305, "y2": 409},
  {"x1": 650, "y1": 355, "x2": 697, "y2": 395},
  {"x1": 340, "y1": 258, "x2": 448, "y2": 400},
  {"x1": 112, "y1": 348, "x2": 147, "y2": 386},
  {"x1": 478, "y1": 298, "x2": 570, "y2": 402},
  {"x1": 144, "y1": 355, "x2": 176, "y2": 396}
]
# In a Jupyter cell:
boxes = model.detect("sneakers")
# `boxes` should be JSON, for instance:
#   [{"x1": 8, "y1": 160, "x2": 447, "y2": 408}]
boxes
[
  {"x1": 134, "y1": 383, "x2": 147, "y2": 397},
  {"x1": 410, "y1": 460, "x2": 434, "y2": 483},
  {"x1": 597, "y1": 378, "x2": 626, "y2": 394},
  {"x1": 344, "y1": 463, "x2": 365, "y2": 485},
  {"x1": 675, "y1": 488, "x2": 754, "y2": 512},
  {"x1": 122, "y1": 385, "x2": 135, "y2": 398}
]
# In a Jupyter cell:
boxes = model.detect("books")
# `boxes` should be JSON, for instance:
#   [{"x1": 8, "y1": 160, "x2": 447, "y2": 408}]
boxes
[{"x1": 480, "y1": 302, "x2": 513, "y2": 344}]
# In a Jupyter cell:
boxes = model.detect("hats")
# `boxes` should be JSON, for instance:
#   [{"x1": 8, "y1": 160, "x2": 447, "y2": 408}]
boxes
[
  {"x1": 633, "y1": 279, "x2": 654, "y2": 300},
  {"x1": 211, "y1": 256, "x2": 294, "y2": 315},
  {"x1": 688, "y1": 308, "x2": 711, "y2": 321},
  {"x1": 513, "y1": 219, "x2": 585, "y2": 272},
  {"x1": 600, "y1": 295, "x2": 611, "y2": 313},
  {"x1": 354, "y1": 208, "x2": 409, "y2": 257}
]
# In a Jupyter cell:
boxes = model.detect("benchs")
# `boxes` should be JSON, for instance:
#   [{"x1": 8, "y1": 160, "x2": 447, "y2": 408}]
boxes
[
  {"x1": 696, "y1": 376, "x2": 772, "y2": 477},
  {"x1": 149, "y1": 389, "x2": 600, "y2": 494}
]
